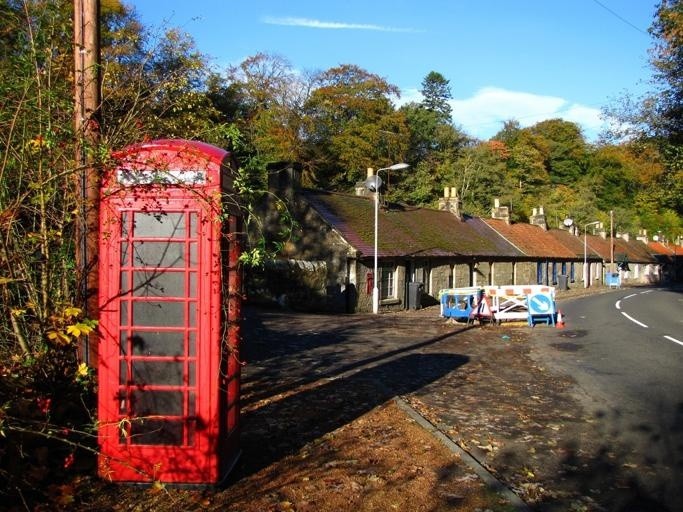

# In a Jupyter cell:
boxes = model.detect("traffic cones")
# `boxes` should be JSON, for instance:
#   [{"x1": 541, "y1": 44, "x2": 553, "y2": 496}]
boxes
[{"x1": 555, "y1": 309, "x2": 565, "y2": 330}]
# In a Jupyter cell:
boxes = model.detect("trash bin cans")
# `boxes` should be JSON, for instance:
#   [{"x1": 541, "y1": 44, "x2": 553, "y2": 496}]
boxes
[
  {"x1": 558, "y1": 275, "x2": 569, "y2": 290},
  {"x1": 408, "y1": 282, "x2": 424, "y2": 309}
]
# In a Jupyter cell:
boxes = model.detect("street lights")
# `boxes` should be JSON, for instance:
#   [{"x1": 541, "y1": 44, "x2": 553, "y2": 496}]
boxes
[
  {"x1": 584, "y1": 220, "x2": 600, "y2": 288},
  {"x1": 372, "y1": 162, "x2": 411, "y2": 315}
]
